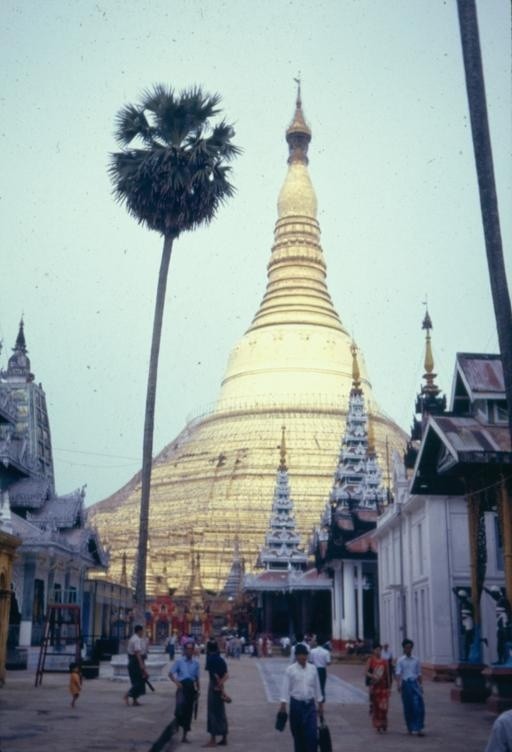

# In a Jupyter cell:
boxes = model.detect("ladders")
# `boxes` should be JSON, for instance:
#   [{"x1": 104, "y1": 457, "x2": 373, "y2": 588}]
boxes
[{"x1": 35, "y1": 604, "x2": 81, "y2": 687}]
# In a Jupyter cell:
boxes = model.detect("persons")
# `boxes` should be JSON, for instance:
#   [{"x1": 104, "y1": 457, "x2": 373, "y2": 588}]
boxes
[
  {"x1": 485, "y1": 709, "x2": 512, "y2": 752},
  {"x1": 458, "y1": 585, "x2": 512, "y2": 665},
  {"x1": 396, "y1": 638, "x2": 427, "y2": 736},
  {"x1": 365, "y1": 644, "x2": 389, "y2": 732},
  {"x1": 69, "y1": 662, "x2": 81, "y2": 708},
  {"x1": 382, "y1": 644, "x2": 395, "y2": 689},
  {"x1": 123, "y1": 625, "x2": 149, "y2": 706},
  {"x1": 165, "y1": 627, "x2": 332, "y2": 752}
]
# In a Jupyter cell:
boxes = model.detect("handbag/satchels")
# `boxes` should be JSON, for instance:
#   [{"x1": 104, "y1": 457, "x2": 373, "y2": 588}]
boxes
[{"x1": 318, "y1": 724, "x2": 332, "y2": 752}]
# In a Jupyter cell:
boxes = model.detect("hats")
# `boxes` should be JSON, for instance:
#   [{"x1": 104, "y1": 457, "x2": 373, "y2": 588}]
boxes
[{"x1": 295, "y1": 645, "x2": 309, "y2": 654}]
